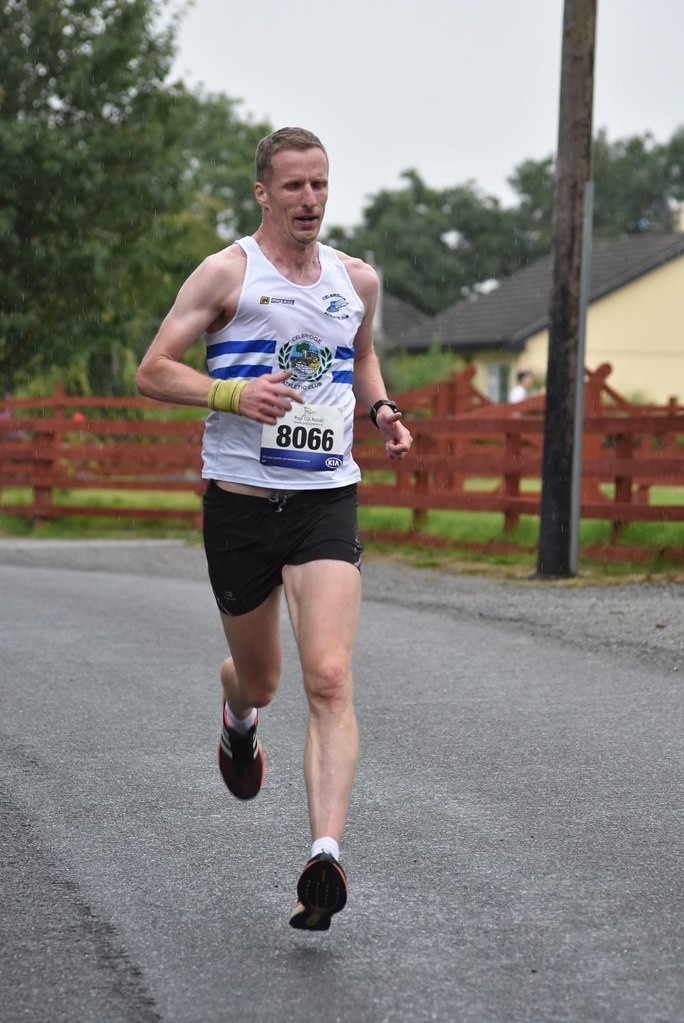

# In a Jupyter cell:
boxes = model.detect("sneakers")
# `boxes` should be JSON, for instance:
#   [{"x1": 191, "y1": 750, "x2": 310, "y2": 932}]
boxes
[
  {"x1": 287, "y1": 853, "x2": 348, "y2": 932},
  {"x1": 217, "y1": 691, "x2": 266, "y2": 802}
]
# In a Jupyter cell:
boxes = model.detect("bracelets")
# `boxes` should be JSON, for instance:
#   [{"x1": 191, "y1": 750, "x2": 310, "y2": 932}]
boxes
[{"x1": 370, "y1": 400, "x2": 399, "y2": 430}]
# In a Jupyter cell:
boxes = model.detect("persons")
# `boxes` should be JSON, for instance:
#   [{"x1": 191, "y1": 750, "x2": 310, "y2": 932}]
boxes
[
  {"x1": 136, "y1": 126, "x2": 412, "y2": 930},
  {"x1": 508, "y1": 372, "x2": 534, "y2": 404}
]
